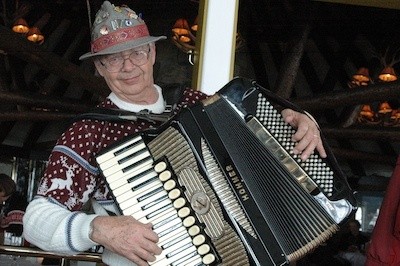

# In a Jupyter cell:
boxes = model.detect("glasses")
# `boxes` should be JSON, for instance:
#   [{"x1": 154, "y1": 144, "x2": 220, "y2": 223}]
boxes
[{"x1": 96, "y1": 43, "x2": 151, "y2": 72}]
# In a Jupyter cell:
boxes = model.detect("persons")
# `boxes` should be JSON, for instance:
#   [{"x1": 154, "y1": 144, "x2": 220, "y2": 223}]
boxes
[
  {"x1": 0, "y1": 173, "x2": 29, "y2": 237},
  {"x1": 22, "y1": 1, "x2": 328, "y2": 266}
]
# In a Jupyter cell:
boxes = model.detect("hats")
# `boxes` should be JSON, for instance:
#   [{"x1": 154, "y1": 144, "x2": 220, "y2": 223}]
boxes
[{"x1": 78, "y1": 0, "x2": 167, "y2": 61}]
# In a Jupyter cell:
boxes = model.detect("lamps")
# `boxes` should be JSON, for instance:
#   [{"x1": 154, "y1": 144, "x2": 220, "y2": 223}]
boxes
[
  {"x1": 350, "y1": 67, "x2": 397, "y2": 88},
  {"x1": 170, "y1": 17, "x2": 198, "y2": 65},
  {"x1": 12, "y1": 18, "x2": 44, "y2": 45}
]
